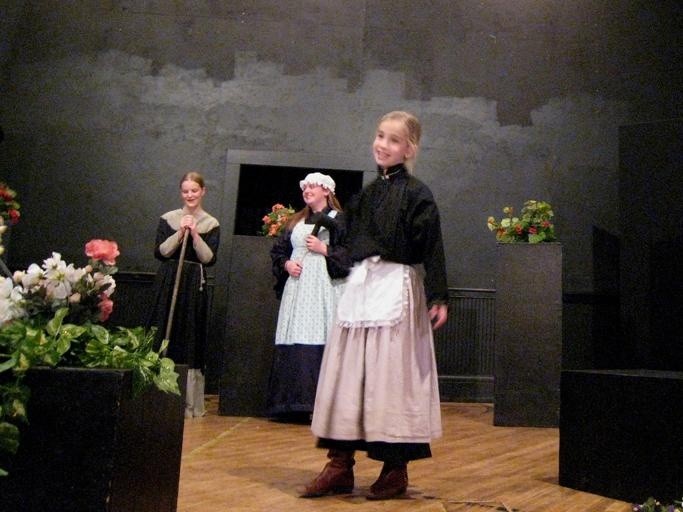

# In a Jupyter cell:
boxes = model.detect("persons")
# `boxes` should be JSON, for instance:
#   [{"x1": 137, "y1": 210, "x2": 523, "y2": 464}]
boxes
[
  {"x1": 143, "y1": 172, "x2": 220, "y2": 421},
  {"x1": 260, "y1": 170, "x2": 349, "y2": 422},
  {"x1": 296, "y1": 109, "x2": 451, "y2": 500}
]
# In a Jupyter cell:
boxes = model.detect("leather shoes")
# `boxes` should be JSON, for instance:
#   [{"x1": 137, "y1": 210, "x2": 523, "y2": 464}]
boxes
[
  {"x1": 365, "y1": 461, "x2": 410, "y2": 501},
  {"x1": 294, "y1": 454, "x2": 355, "y2": 499}
]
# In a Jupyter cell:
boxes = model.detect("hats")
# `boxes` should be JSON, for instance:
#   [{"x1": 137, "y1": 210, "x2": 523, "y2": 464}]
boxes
[{"x1": 297, "y1": 171, "x2": 336, "y2": 196}]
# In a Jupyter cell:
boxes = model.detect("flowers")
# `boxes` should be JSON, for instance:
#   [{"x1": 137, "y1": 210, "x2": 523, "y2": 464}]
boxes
[
  {"x1": 257, "y1": 203, "x2": 297, "y2": 238},
  {"x1": 485, "y1": 199, "x2": 557, "y2": 244},
  {"x1": 0, "y1": 182, "x2": 181, "y2": 481}
]
